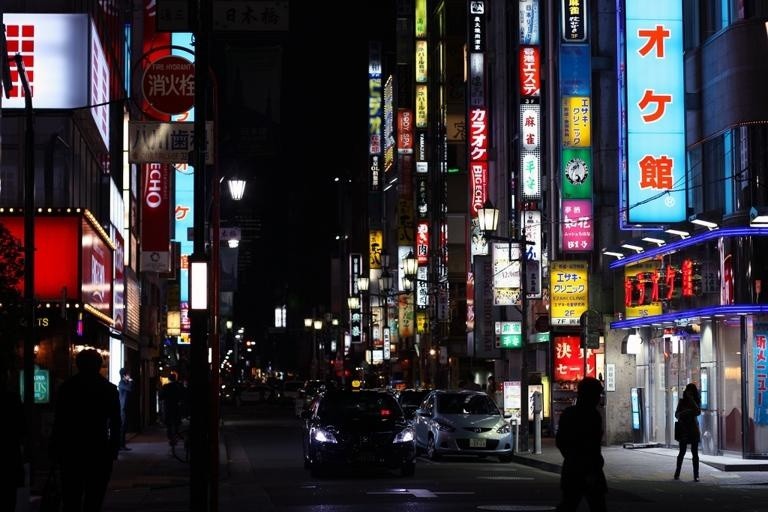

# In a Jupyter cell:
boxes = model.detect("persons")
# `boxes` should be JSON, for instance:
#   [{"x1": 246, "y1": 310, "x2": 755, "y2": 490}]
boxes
[
  {"x1": 55, "y1": 349, "x2": 123, "y2": 511},
  {"x1": 118, "y1": 368, "x2": 137, "y2": 451},
  {"x1": 160, "y1": 375, "x2": 191, "y2": 458},
  {"x1": 675, "y1": 383, "x2": 702, "y2": 482},
  {"x1": 556, "y1": 378, "x2": 610, "y2": 511}
]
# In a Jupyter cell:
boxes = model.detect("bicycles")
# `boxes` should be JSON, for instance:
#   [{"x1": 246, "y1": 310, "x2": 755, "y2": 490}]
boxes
[{"x1": 169, "y1": 420, "x2": 194, "y2": 461}]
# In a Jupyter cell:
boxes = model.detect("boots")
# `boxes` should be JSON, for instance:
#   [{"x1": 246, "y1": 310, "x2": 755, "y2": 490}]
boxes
[
  {"x1": 692, "y1": 457, "x2": 699, "y2": 482},
  {"x1": 674, "y1": 456, "x2": 684, "y2": 480}
]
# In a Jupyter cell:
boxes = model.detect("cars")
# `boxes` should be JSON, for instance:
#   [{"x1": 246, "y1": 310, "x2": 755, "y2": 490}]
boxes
[
  {"x1": 241, "y1": 385, "x2": 280, "y2": 402},
  {"x1": 284, "y1": 380, "x2": 398, "y2": 423},
  {"x1": 303, "y1": 390, "x2": 417, "y2": 479},
  {"x1": 398, "y1": 388, "x2": 514, "y2": 463}
]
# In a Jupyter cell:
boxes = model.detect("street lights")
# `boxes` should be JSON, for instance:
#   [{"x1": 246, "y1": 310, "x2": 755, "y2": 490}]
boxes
[
  {"x1": 478, "y1": 199, "x2": 535, "y2": 453},
  {"x1": 305, "y1": 317, "x2": 350, "y2": 379},
  {"x1": 358, "y1": 266, "x2": 391, "y2": 382},
  {"x1": 200, "y1": 175, "x2": 251, "y2": 511},
  {"x1": 405, "y1": 251, "x2": 444, "y2": 384}
]
